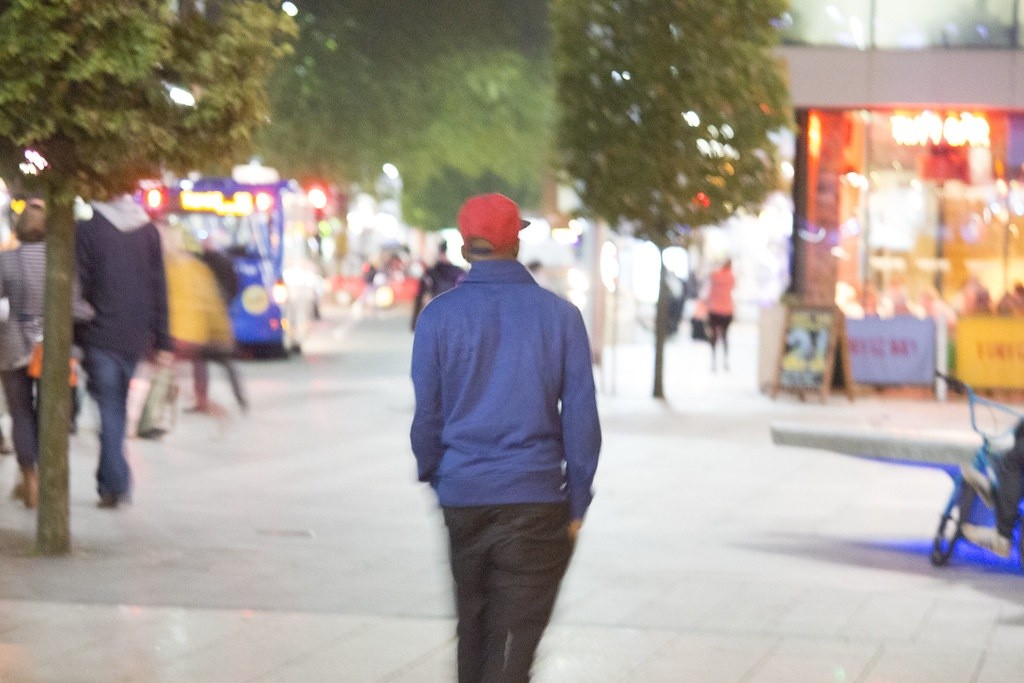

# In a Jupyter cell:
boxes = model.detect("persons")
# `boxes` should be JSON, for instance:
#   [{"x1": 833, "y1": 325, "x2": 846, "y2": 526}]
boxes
[
  {"x1": 703, "y1": 259, "x2": 736, "y2": 369},
  {"x1": 73, "y1": 170, "x2": 174, "y2": 509},
  {"x1": 138, "y1": 222, "x2": 251, "y2": 438},
  {"x1": 963, "y1": 416, "x2": 1024, "y2": 557},
  {"x1": 0, "y1": 202, "x2": 95, "y2": 508},
  {"x1": 365, "y1": 237, "x2": 466, "y2": 332},
  {"x1": 411, "y1": 190, "x2": 601, "y2": 683}
]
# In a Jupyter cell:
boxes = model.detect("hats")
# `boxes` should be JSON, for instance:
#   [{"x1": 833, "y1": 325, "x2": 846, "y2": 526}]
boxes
[{"x1": 457, "y1": 194, "x2": 533, "y2": 254}]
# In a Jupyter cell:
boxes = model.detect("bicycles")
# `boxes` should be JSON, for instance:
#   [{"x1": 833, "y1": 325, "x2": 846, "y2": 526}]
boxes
[{"x1": 928, "y1": 369, "x2": 1024, "y2": 569}]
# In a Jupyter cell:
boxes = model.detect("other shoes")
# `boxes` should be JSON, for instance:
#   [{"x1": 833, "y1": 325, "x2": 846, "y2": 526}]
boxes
[
  {"x1": 11, "y1": 480, "x2": 38, "y2": 510},
  {"x1": 96, "y1": 488, "x2": 132, "y2": 509}
]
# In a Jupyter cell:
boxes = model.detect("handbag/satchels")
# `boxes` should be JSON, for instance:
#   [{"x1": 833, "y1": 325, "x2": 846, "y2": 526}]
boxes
[
  {"x1": 689, "y1": 316, "x2": 716, "y2": 344},
  {"x1": 135, "y1": 354, "x2": 180, "y2": 438},
  {"x1": 26, "y1": 343, "x2": 79, "y2": 389}
]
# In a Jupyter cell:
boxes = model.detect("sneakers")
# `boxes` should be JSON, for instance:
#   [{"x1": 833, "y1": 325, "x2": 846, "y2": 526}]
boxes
[
  {"x1": 959, "y1": 462, "x2": 997, "y2": 511},
  {"x1": 960, "y1": 522, "x2": 1012, "y2": 560}
]
